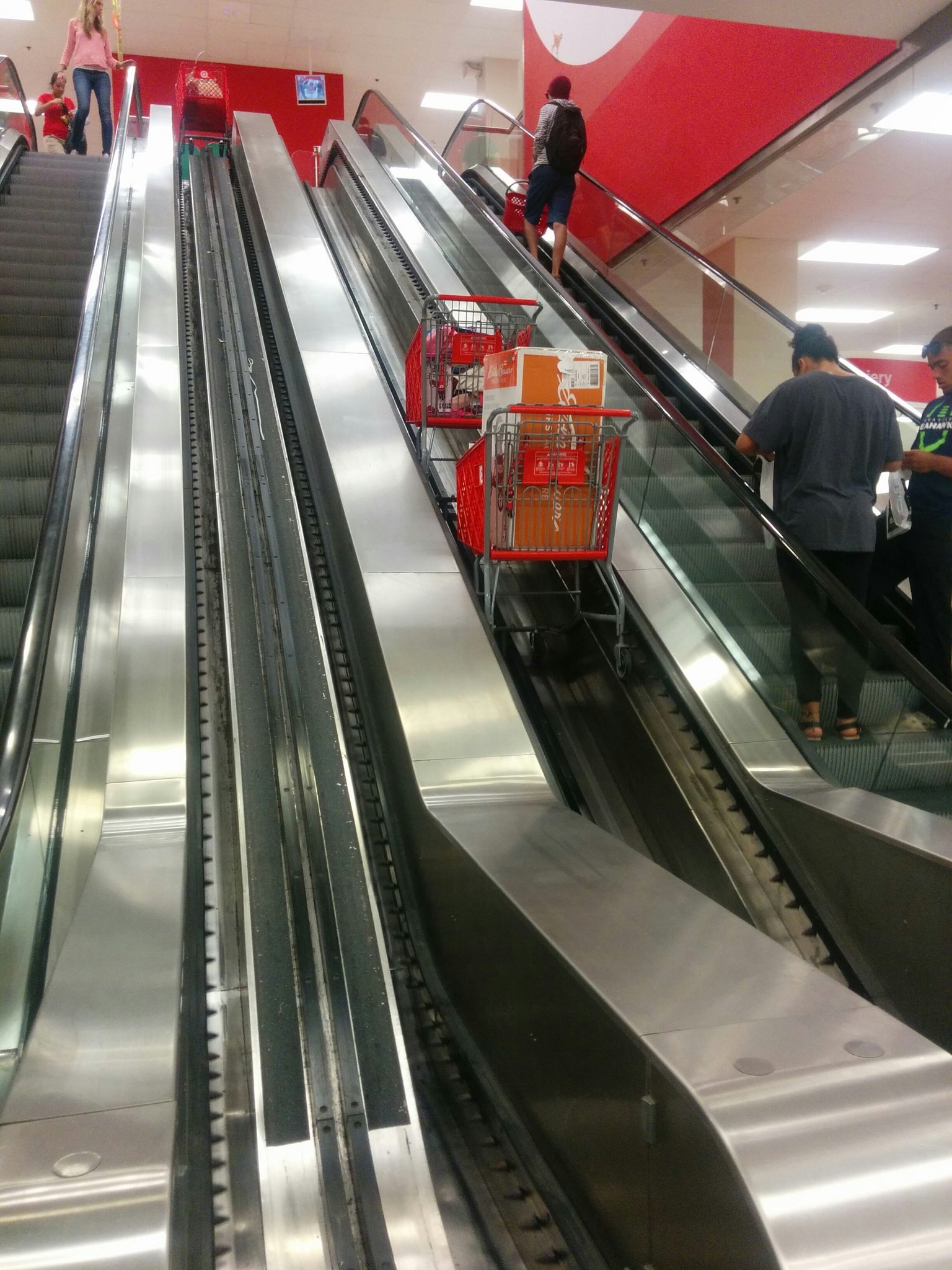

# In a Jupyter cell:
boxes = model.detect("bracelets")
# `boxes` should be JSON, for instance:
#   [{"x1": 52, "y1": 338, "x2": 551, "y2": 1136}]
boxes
[{"x1": 57, "y1": 70, "x2": 63, "y2": 73}]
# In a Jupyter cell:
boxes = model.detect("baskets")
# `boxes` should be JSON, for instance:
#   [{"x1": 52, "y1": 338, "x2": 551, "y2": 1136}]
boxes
[{"x1": 502, "y1": 181, "x2": 551, "y2": 238}]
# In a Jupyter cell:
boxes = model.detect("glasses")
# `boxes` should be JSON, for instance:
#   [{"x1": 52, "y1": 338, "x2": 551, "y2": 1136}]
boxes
[{"x1": 920, "y1": 340, "x2": 950, "y2": 357}]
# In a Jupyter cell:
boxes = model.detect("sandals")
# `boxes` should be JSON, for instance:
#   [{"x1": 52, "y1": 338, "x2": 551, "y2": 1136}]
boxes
[
  {"x1": 838, "y1": 718, "x2": 862, "y2": 742},
  {"x1": 798, "y1": 721, "x2": 821, "y2": 740}
]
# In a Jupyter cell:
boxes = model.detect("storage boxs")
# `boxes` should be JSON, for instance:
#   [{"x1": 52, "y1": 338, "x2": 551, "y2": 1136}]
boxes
[{"x1": 481, "y1": 343, "x2": 605, "y2": 551}]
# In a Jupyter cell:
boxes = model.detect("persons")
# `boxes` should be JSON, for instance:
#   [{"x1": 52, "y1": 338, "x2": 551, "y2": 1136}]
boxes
[
  {"x1": 522, "y1": 76, "x2": 588, "y2": 287},
  {"x1": 65, "y1": 127, "x2": 88, "y2": 156},
  {"x1": 297, "y1": 79, "x2": 324, "y2": 99},
  {"x1": 2, "y1": 68, "x2": 32, "y2": 151},
  {"x1": 355, "y1": 116, "x2": 387, "y2": 161},
  {"x1": 33, "y1": 71, "x2": 76, "y2": 156},
  {"x1": 733, "y1": 322, "x2": 906, "y2": 742},
  {"x1": 865, "y1": 326, "x2": 952, "y2": 734},
  {"x1": 57, "y1": 0, "x2": 138, "y2": 159}
]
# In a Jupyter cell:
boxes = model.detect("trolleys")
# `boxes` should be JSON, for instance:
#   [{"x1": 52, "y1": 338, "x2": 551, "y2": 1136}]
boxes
[
  {"x1": 406, "y1": 293, "x2": 639, "y2": 673},
  {"x1": 174, "y1": 61, "x2": 232, "y2": 158}
]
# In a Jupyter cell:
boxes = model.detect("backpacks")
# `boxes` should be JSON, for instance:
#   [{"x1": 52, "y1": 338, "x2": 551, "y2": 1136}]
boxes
[{"x1": 545, "y1": 100, "x2": 586, "y2": 176}]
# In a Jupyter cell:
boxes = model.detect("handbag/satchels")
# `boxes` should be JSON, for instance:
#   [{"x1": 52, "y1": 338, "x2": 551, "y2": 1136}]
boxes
[{"x1": 181, "y1": 50, "x2": 225, "y2": 100}]
